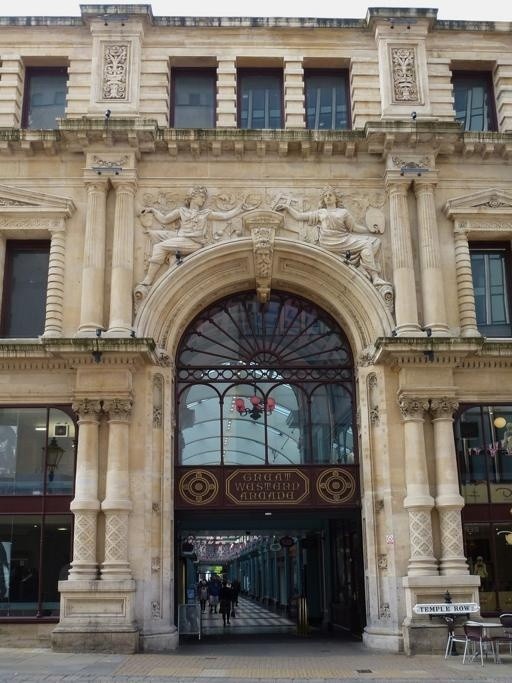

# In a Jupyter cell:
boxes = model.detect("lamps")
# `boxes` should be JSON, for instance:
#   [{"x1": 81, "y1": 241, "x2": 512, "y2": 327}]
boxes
[{"x1": 235, "y1": 382, "x2": 276, "y2": 420}]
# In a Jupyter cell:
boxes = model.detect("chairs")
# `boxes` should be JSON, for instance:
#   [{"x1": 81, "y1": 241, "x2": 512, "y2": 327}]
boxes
[{"x1": 444, "y1": 611, "x2": 512, "y2": 667}]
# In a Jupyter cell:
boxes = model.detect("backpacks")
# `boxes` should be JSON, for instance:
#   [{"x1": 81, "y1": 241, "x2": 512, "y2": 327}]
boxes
[{"x1": 200, "y1": 586, "x2": 208, "y2": 600}]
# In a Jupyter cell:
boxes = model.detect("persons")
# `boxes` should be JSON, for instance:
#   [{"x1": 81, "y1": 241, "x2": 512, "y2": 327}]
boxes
[
  {"x1": 504, "y1": 422, "x2": 512, "y2": 449},
  {"x1": 134, "y1": 187, "x2": 249, "y2": 286},
  {"x1": 274, "y1": 189, "x2": 391, "y2": 287},
  {"x1": 22, "y1": 568, "x2": 33, "y2": 586},
  {"x1": 473, "y1": 556, "x2": 488, "y2": 593},
  {"x1": 197, "y1": 573, "x2": 241, "y2": 626}
]
